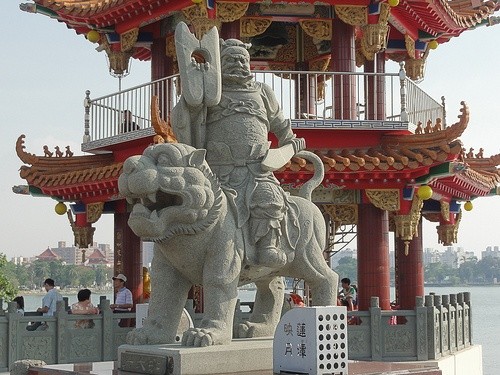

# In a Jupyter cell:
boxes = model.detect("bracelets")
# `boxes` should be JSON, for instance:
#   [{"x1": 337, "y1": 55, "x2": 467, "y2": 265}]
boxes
[{"x1": 117, "y1": 304, "x2": 119, "y2": 308}]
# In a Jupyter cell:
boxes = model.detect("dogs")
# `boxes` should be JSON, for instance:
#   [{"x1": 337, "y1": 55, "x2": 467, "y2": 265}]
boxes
[
  {"x1": 433, "y1": 118, "x2": 441, "y2": 133},
  {"x1": 462, "y1": 147, "x2": 466, "y2": 159},
  {"x1": 54, "y1": 146, "x2": 63, "y2": 158},
  {"x1": 415, "y1": 120, "x2": 422, "y2": 135},
  {"x1": 466, "y1": 147, "x2": 474, "y2": 158},
  {"x1": 423, "y1": 119, "x2": 432, "y2": 134},
  {"x1": 65, "y1": 145, "x2": 73, "y2": 158},
  {"x1": 43, "y1": 145, "x2": 53, "y2": 158},
  {"x1": 475, "y1": 148, "x2": 484, "y2": 159}
]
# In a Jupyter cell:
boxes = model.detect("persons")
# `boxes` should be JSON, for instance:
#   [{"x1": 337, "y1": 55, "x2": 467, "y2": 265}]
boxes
[
  {"x1": 121, "y1": 109, "x2": 139, "y2": 134},
  {"x1": 13, "y1": 296, "x2": 25, "y2": 317},
  {"x1": 389, "y1": 306, "x2": 396, "y2": 324},
  {"x1": 37, "y1": 278, "x2": 63, "y2": 327},
  {"x1": 110, "y1": 274, "x2": 133, "y2": 327},
  {"x1": 291, "y1": 292, "x2": 304, "y2": 305},
  {"x1": 143, "y1": 266, "x2": 151, "y2": 300},
  {"x1": 71, "y1": 289, "x2": 99, "y2": 328},
  {"x1": 192, "y1": 38, "x2": 306, "y2": 269},
  {"x1": 337, "y1": 278, "x2": 357, "y2": 324}
]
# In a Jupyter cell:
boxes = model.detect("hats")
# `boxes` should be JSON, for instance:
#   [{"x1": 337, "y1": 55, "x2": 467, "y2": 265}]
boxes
[{"x1": 112, "y1": 274, "x2": 127, "y2": 282}]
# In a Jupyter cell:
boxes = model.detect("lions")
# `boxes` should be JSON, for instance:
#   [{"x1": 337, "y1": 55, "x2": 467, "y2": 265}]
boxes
[{"x1": 118, "y1": 141, "x2": 340, "y2": 347}]
